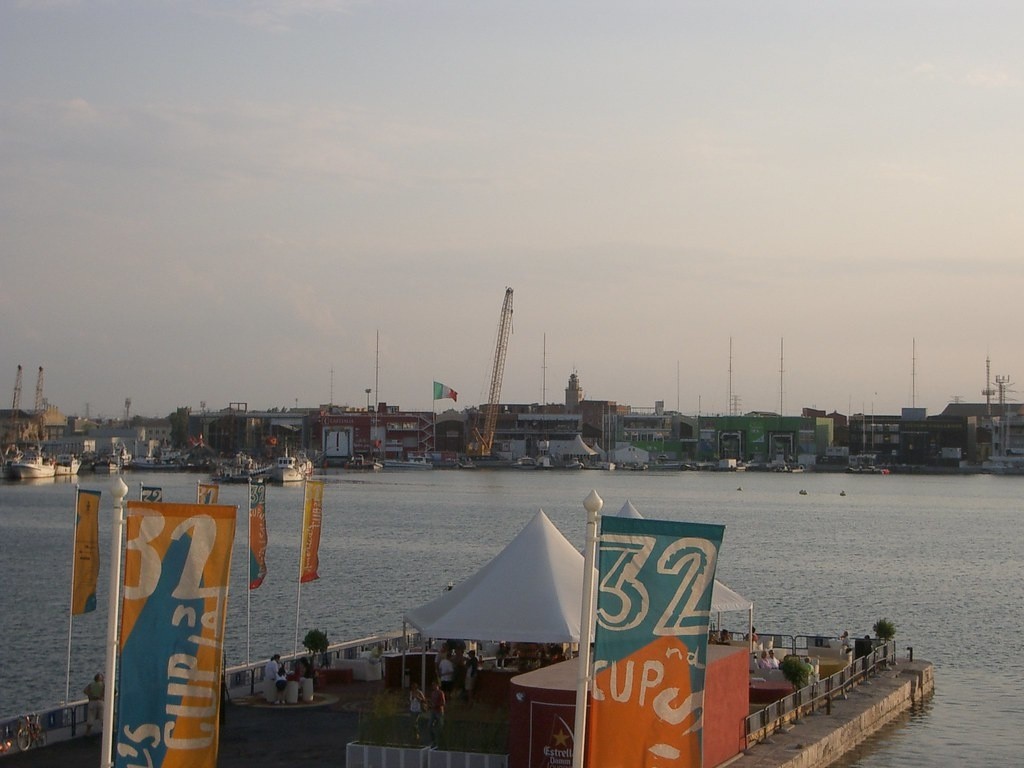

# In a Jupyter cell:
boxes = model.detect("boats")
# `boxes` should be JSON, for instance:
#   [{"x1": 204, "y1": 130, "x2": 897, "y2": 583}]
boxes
[{"x1": 1, "y1": 444, "x2": 584, "y2": 488}]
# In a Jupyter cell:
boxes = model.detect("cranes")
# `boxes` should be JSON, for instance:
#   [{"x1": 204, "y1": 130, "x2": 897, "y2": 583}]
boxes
[
  {"x1": 471, "y1": 287, "x2": 514, "y2": 448},
  {"x1": 9, "y1": 364, "x2": 47, "y2": 441}
]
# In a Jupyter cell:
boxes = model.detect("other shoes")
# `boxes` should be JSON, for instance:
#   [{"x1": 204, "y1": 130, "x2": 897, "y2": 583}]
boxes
[
  {"x1": 274, "y1": 701, "x2": 280, "y2": 704},
  {"x1": 282, "y1": 701, "x2": 285, "y2": 703}
]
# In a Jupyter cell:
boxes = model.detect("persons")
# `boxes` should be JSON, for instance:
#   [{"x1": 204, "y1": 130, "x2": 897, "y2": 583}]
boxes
[
  {"x1": 746, "y1": 627, "x2": 758, "y2": 642},
  {"x1": 435, "y1": 639, "x2": 478, "y2": 706},
  {"x1": 759, "y1": 650, "x2": 781, "y2": 670},
  {"x1": 372, "y1": 645, "x2": 385, "y2": 662},
  {"x1": 410, "y1": 682, "x2": 444, "y2": 749},
  {"x1": 804, "y1": 658, "x2": 815, "y2": 676},
  {"x1": 497, "y1": 642, "x2": 595, "y2": 665},
  {"x1": 299, "y1": 656, "x2": 315, "y2": 696},
  {"x1": 709, "y1": 629, "x2": 731, "y2": 644},
  {"x1": 266, "y1": 654, "x2": 287, "y2": 704},
  {"x1": 865, "y1": 635, "x2": 872, "y2": 657},
  {"x1": 839, "y1": 632, "x2": 850, "y2": 649},
  {"x1": 84, "y1": 673, "x2": 106, "y2": 737}
]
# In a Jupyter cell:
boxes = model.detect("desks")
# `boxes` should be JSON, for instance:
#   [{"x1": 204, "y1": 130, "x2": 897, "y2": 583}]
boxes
[
  {"x1": 462, "y1": 668, "x2": 519, "y2": 707},
  {"x1": 750, "y1": 682, "x2": 794, "y2": 703},
  {"x1": 382, "y1": 652, "x2": 438, "y2": 689},
  {"x1": 812, "y1": 657, "x2": 847, "y2": 679}
]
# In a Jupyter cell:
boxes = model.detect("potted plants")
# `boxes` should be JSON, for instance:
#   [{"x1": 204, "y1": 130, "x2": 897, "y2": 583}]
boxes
[
  {"x1": 346, "y1": 689, "x2": 435, "y2": 768},
  {"x1": 426, "y1": 694, "x2": 510, "y2": 767}
]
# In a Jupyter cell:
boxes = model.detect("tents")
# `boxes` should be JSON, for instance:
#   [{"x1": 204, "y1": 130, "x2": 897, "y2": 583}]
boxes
[
  {"x1": 582, "y1": 499, "x2": 753, "y2": 654},
  {"x1": 402, "y1": 508, "x2": 599, "y2": 695}
]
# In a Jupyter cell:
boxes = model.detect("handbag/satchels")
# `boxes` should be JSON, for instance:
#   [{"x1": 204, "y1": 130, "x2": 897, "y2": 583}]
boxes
[{"x1": 421, "y1": 700, "x2": 429, "y2": 712}]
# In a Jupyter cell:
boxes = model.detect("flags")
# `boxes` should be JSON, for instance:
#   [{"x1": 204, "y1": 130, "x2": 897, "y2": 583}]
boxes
[
  {"x1": 433, "y1": 381, "x2": 458, "y2": 402},
  {"x1": 587, "y1": 516, "x2": 726, "y2": 768},
  {"x1": 73, "y1": 489, "x2": 101, "y2": 613},
  {"x1": 301, "y1": 480, "x2": 324, "y2": 583},
  {"x1": 114, "y1": 501, "x2": 237, "y2": 768},
  {"x1": 250, "y1": 482, "x2": 268, "y2": 589},
  {"x1": 199, "y1": 484, "x2": 219, "y2": 505},
  {"x1": 142, "y1": 487, "x2": 162, "y2": 503}
]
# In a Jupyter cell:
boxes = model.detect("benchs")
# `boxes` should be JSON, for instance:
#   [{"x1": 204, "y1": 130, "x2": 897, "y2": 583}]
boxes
[
  {"x1": 335, "y1": 658, "x2": 382, "y2": 682},
  {"x1": 263, "y1": 677, "x2": 313, "y2": 703},
  {"x1": 314, "y1": 668, "x2": 353, "y2": 683},
  {"x1": 757, "y1": 658, "x2": 820, "y2": 685},
  {"x1": 753, "y1": 647, "x2": 787, "y2": 663}
]
